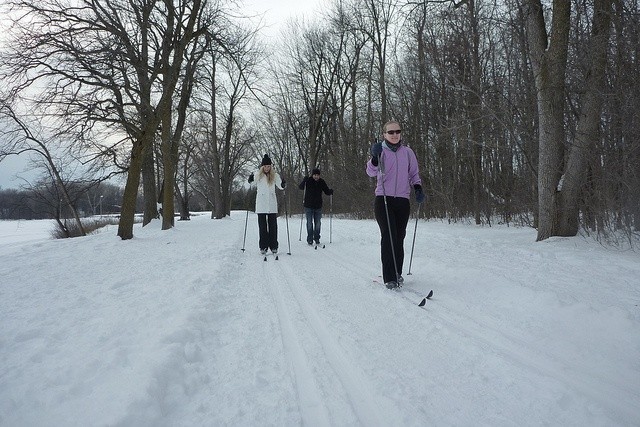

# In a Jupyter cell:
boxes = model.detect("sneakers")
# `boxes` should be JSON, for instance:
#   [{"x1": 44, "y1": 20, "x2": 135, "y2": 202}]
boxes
[
  {"x1": 397, "y1": 274, "x2": 404, "y2": 283},
  {"x1": 270, "y1": 248, "x2": 277, "y2": 253},
  {"x1": 260, "y1": 248, "x2": 268, "y2": 254},
  {"x1": 315, "y1": 238, "x2": 320, "y2": 243},
  {"x1": 385, "y1": 281, "x2": 397, "y2": 289},
  {"x1": 308, "y1": 239, "x2": 313, "y2": 244}
]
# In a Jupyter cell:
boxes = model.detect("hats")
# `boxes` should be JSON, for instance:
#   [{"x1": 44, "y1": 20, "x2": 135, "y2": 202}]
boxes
[
  {"x1": 311, "y1": 168, "x2": 320, "y2": 175},
  {"x1": 262, "y1": 154, "x2": 271, "y2": 165}
]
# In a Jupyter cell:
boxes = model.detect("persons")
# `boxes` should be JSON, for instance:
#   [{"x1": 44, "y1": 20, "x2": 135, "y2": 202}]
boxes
[
  {"x1": 248, "y1": 153, "x2": 287, "y2": 254},
  {"x1": 298, "y1": 168, "x2": 334, "y2": 245},
  {"x1": 365, "y1": 120, "x2": 425, "y2": 290}
]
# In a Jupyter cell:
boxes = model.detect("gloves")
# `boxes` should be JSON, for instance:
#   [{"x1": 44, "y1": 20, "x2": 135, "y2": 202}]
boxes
[
  {"x1": 281, "y1": 178, "x2": 286, "y2": 188},
  {"x1": 248, "y1": 175, "x2": 254, "y2": 183},
  {"x1": 371, "y1": 142, "x2": 382, "y2": 160},
  {"x1": 415, "y1": 189, "x2": 425, "y2": 202}
]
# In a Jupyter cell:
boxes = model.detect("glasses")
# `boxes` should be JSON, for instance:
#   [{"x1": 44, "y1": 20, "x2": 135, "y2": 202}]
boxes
[{"x1": 384, "y1": 130, "x2": 402, "y2": 134}]
